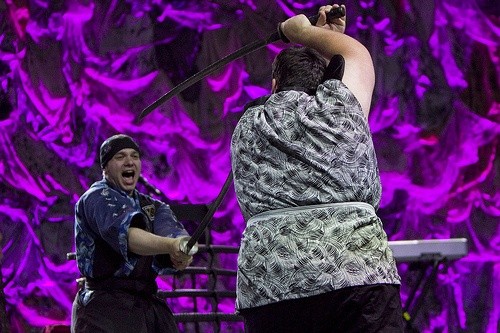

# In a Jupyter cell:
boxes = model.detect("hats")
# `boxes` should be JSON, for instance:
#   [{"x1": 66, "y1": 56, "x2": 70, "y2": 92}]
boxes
[{"x1": 100, "y1": 134, "x2": 142, "y2": 168}]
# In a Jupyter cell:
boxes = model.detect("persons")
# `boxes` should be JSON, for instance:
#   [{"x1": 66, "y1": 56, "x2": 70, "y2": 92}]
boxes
[
  {"x1": 72, "y1": 134, "x2": 193, "y2": 333},
  {"x1": 230, "y1": 4, "x2": 405, "y2": 333}
]
